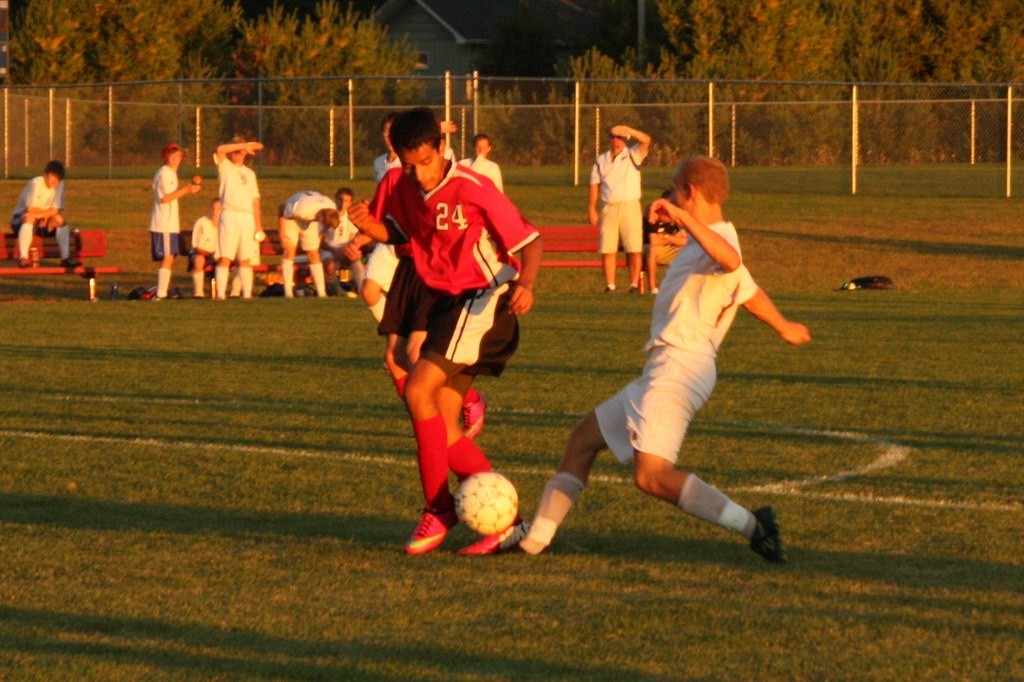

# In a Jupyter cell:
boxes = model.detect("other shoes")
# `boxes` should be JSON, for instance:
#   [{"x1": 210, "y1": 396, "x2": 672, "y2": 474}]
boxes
[
  {"x1": 16, "y1": 256, "x2": 30, "y2": 266},
  {"x1": 61, "y1": 257, "x2": 81, "y2": 266}
]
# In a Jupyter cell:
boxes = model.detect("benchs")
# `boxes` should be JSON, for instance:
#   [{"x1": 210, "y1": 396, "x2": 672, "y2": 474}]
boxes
[
  {"x1": 535, "y1": 226, "x2": 668, "y2": 295},
  {"x1": 202, "y1": 229, "x2": 310, "y2": 271},
  {"x1": 0, "y1": 229, "x2": 120, "y2": 302}
]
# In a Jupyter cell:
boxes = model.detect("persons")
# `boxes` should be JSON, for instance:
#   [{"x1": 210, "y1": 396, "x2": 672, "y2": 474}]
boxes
[
  {"x1": 213, "y1": 136, "x2": 267, "y2": 299},
  {"x1": 9, "y1": 160, "x2": 80, "y2": 268},
  {"x1": 278, "y1": 109, "x2": 505, "y2": 369},
  {"x1": 191, "y1": 197, "x2": 242, "y2": 297},
  {"x1": 520, "y1": 149, "x2": 809, "y2": 566},
  {"x1": 588, "y1": 124, "x2": 651, "y2": 293},
  {"x1": 643, "y1": 189, "x2": 688, "y2": 295},
  {"x1": 149, "y1": 142, "x2": 203, "y2": 299},
  {"x1": 344, "y1": 198, "x2": 488, "y2": 439},
  {"x1": 343, "y1": 110, "x2": 538, "y2": 551}
]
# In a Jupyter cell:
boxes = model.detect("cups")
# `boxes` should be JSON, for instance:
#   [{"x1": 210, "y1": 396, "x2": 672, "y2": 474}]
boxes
[{"x1": 255, "y1": 231, "x2": 265, "y2": 242}]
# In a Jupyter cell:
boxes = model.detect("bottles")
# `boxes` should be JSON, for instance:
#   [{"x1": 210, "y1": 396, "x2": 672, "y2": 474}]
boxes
[
  {"x1": 191, "y1": 176, "x2": 202, "y2": 185},
  {"x1": 111, "y1": 283, "x2": 119, "y2": 301},
  {"x1": 30, "y1": 247, "x2": 39, "y2": 268}
]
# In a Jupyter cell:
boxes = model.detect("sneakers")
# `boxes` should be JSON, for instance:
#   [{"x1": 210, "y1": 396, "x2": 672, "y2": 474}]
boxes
[
  {"x1": 748, "y1": 506, "x2": 788, "y2": 564},
  {"x1": 457, "y1": 519, "x2": 532, "y2": 557},
  {"x1": 496, "y1": 544, "x2": 551, "y2": 556},
  {"x1": 462, "y1": 393, "x2": 487, "y2": 439},
  {"x1": 405, "y1": 496, "x2": 457, "y2": 555}
]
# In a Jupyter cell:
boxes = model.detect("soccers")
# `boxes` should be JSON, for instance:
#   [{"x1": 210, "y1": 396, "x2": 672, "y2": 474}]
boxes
[{"x1": 453, "y1": 471, "x2": 519, "y2": 536}]
